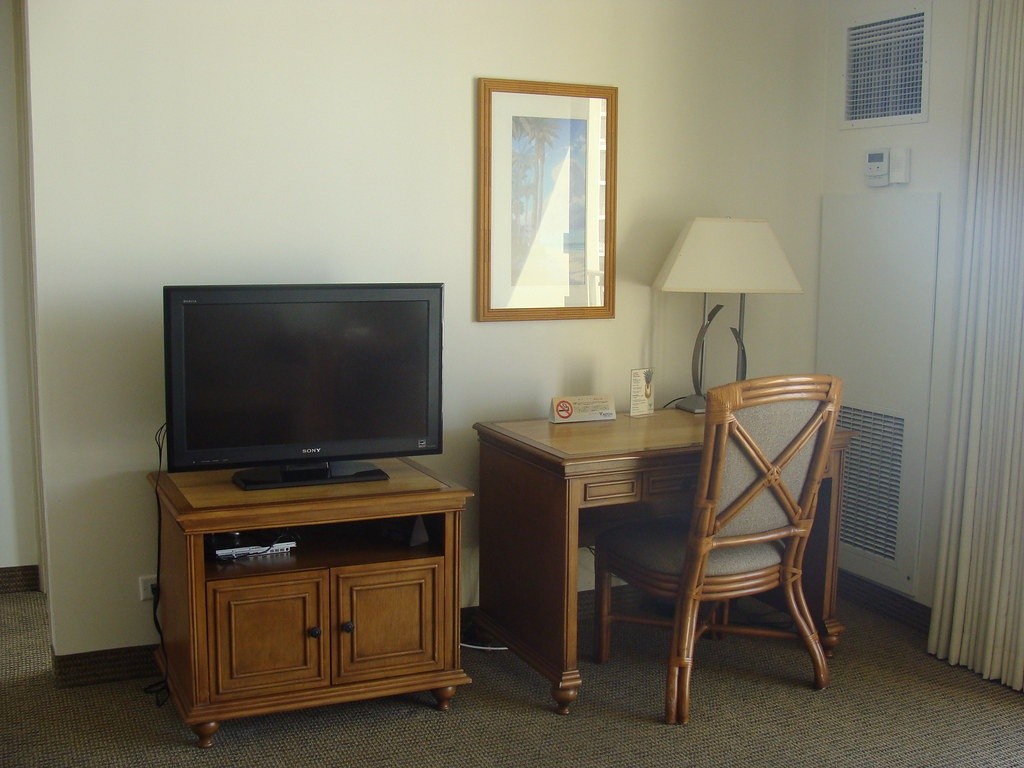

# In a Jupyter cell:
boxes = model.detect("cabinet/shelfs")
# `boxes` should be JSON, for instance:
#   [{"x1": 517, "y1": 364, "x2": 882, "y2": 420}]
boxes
[{"x1": 148, "y1": 455, "x2": 475, "y2": 748}]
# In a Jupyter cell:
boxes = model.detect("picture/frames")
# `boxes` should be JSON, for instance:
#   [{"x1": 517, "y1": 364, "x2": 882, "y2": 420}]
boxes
[{"x1": 475, "y1": 78, "x2": 618, "y2": 320}]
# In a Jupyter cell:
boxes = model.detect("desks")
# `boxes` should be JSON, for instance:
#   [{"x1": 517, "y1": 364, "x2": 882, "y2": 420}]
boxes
[{"x1": 470, "y1": 406, "x2": 864, "y2": 717}]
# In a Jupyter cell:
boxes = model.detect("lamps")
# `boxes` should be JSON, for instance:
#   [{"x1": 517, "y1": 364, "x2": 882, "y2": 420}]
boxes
[{"x1": 649, "y1": 215, "x2": 804, "y2": 415}]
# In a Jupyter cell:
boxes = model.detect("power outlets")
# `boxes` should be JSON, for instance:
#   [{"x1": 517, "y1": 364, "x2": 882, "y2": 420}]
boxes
[{"x1": 139, "y1": 574, "x2": 156, "y2": 601}]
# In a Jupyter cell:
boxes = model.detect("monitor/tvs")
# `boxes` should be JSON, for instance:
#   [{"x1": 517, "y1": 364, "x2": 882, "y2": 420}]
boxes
[{"x1": 164, "y1": 282, "x2": 444, "y2": 491}]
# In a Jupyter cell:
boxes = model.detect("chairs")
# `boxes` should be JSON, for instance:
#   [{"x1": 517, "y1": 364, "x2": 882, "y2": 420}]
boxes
[{"x1": 593, "y1": 376, "x2": 843, "y2": 730}]
